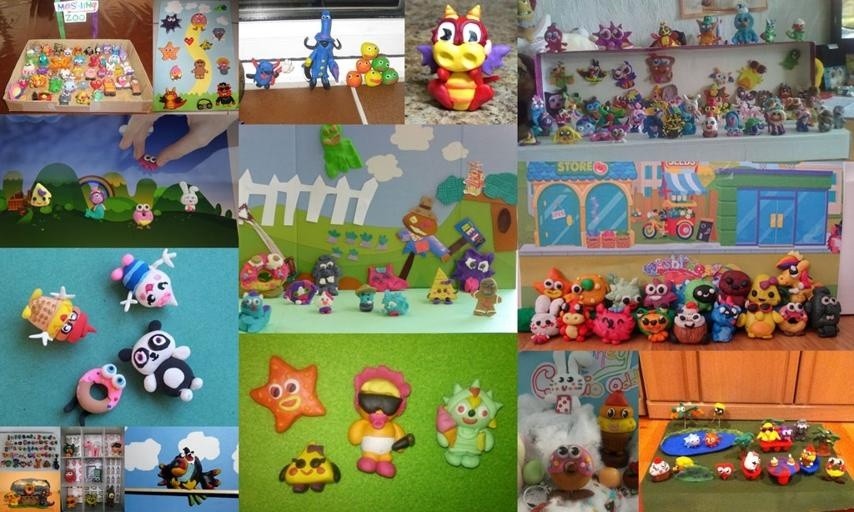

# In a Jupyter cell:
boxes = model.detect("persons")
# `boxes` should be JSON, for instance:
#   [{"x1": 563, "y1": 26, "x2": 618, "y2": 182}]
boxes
[
  {"x1": 117, "y1": 112, "x2": 238, "y2": 168},
  {"x1": 1, "y1": 0, "x2": 239, "y2": 114}
]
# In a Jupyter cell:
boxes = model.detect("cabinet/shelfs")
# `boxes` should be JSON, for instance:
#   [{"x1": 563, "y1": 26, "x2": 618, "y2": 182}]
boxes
[
  {"x1": 61, "y1": 426, "x2": 125, "y2": 511},
  {"x1": 518, "y1": 41, "x2": 851, "y2": 161},
  {"x1": 639, "y1": 350, "x2": 853, "y2": 424}
]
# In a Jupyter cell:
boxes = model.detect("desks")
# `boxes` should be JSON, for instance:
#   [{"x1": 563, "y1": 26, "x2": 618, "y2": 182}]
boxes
[{"x1": 639, "y1": 419, "x2": 854, "y2": 512}]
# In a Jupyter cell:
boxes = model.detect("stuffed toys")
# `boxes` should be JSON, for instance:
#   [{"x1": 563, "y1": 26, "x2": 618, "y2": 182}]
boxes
[{"x1": 240, "y1": 123, "x2": 517, "y2": 335}]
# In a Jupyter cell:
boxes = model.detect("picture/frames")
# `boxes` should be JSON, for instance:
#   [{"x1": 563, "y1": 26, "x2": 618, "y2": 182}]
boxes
[{"x1": 679, "y1": 0, "x2": 769, "y2": 20}]
